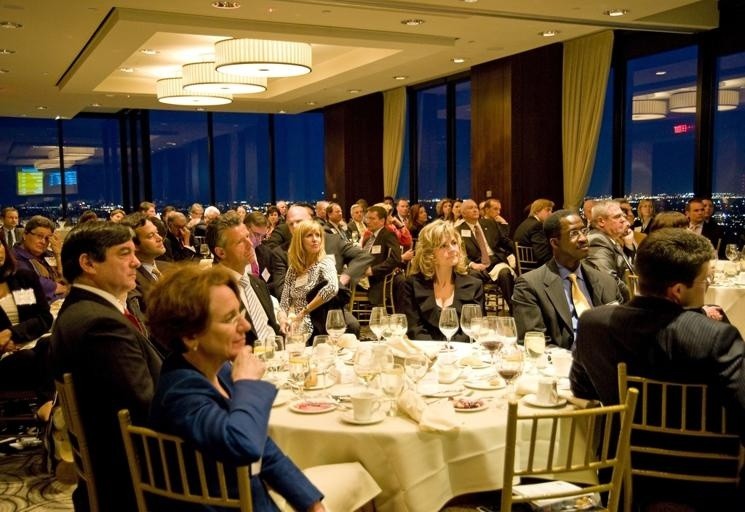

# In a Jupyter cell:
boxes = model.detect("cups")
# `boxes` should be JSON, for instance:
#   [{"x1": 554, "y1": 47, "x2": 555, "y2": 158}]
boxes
[{"x1": 710, "y1": 249, "x2": 719, "y2": 267}]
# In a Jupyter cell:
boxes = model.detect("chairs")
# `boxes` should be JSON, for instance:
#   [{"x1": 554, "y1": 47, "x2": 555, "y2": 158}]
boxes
[
  {"x1": 52, "y1": 372, "x2": 100, "y2": 512},
  {"x1": 443, "y1": 385, "x2": 639, "y2": 512},
  {"x1": 118, "y1": 409, "x2": 255, "y2": 512},
  {"x1": 347, "y1": 240, "x2": 642, "y2": 325},
  {"x1": 616, "y1": 361, "x2": 743, "y2": 512}
]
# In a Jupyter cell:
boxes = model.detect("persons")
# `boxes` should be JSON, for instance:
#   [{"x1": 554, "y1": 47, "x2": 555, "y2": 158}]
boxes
[
  {"x1": 513, "y1": 194, "x2": 733, "y2": 304},
  {"x1": 511, "y1": 209, "x2": 626, "y2": 350},
  {"x1": 568, "y1": 229, "x2": 744, "y2": 512},
  {"x1": 0, "y1": 201, "x2": 335, "y2": 512},
  {"x1": 316, "y1": 196, "x2": 522, "y2": 320},
  {"x1": 145, "y1": 265, "x2": 383, "y2": 512},
  {"x1": 396, "y1": 219, "x2": 487, "y2": 343}
]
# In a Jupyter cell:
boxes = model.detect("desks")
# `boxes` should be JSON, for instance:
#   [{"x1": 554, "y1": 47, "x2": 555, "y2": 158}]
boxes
[{"x1": 252, "y1": 339, "x2": 602, "y2": 512}]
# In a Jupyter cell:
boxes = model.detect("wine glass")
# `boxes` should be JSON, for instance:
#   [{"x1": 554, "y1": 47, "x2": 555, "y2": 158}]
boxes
[
  {"x1": 199, "y1": 243, "x2": 210, "y2": 259},
  {"x1": 56, "y1": 220, "x2": 69, "y2": 229},
  {"x1": 352, "y1": 231, "x2": 358, "y2": 246},
  {"x1": 254, "y1": 303, "x2": 546, "y2": 404},
  {"x1": 721, "y1": 244, "x2": 745, "y2": 284}
]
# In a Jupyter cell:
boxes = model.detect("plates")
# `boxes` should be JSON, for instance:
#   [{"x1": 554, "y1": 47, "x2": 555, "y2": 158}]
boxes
[
  {"x1": 248, "y1": 355, "x2": 575, "y2": 426},
  {"x1": 48, "y1": 299, "x2": 63, "y2": 311}
]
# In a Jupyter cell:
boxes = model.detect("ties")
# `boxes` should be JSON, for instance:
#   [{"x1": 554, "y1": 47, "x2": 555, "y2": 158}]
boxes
[
  {"x1": 251, "y1": 258, "x2": 259, "y2": 273},
  {"x1": 152, "y1": 268, "x2": 161, "y2": 277},
  {"x1": 359, "y1": 221, "x2": 365, "y2": 231},
  {"x1": 365, "y1": 235, "x2": 375, "y2": 252},
  {"x1": 337, "y1": 223, "x2": 348, "y2": 241},
  {"x1": 7, "y1": 229, "x2": 14, "y2": 250},
  {"x1": 473, "y1": 224, "x2": 491, "y2": 264},
  {"x1": 567, "y1": 273, "x2": 591, "y2": 318},
  {"x1": 238, "y1": 275, "x2": 275, "y2": 346}
]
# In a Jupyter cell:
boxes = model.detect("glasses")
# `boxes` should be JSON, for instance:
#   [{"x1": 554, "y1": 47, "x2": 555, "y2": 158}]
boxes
[
  {"x1": 28, "y1": 230, "x2": 47, "y2": 241},
  {"x1": 559, "y1": 226, "x2": 588, "y2": 237}
]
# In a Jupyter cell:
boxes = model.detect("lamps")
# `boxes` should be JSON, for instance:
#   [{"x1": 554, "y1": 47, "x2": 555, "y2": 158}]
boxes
[
  {"x1": 157, "y1": 40, "x2": 312, "y2": 107},
  {"x1": 631, "y1": 90, "x2": 739, "y2": 121}
]
